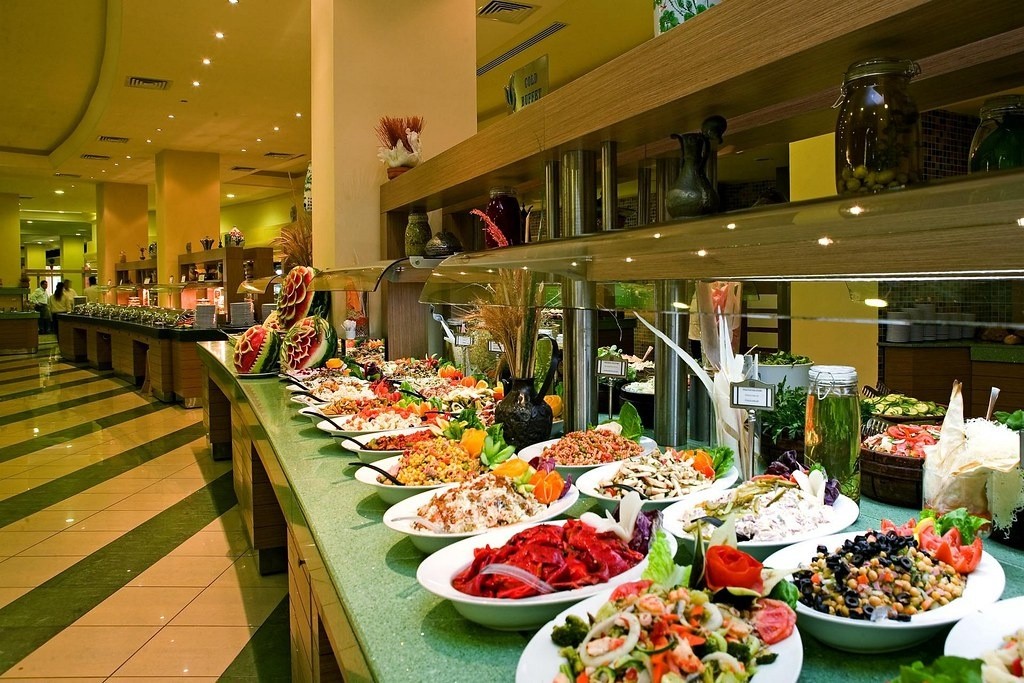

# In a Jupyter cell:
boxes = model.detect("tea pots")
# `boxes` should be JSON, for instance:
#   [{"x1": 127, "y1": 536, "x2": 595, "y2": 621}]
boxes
[{"x1": 666, "y1": 115, "x2": 728, "y2": 220}]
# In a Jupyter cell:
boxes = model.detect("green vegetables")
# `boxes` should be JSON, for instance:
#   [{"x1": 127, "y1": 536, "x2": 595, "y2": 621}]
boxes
[
  {"x1": 343, "y1": 354, "x2": 644, "y2": 489},
  {"x1": 552, "y1": 446, "x2": 992, "y2": 683}
]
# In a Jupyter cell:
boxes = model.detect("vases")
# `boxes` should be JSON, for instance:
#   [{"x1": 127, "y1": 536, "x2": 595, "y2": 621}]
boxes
[
  {"x1": 665, "y1": 131, "x2": 719, "y2": 222},
  {"x1": 304, "y1": 160, "x2": 312, "y2": 214},
  {"x1": 230, "y1": 225, "x2": 246, "y2": 246},
  {"x1": 425, "y1": 232, "x2": 462, "y2": 256},
  {"x1": 485, "y1": 185, "x2": 522, "y2": 250},
  {"x1": 199, "y1": 236, "x2": 214, "y2": 251},
  {"x1": 140, "y1": 248, "x2": 146, "y2": 261},
  {"x1": 386, "y1": 166, "x2": 412, "y2": 181},
  {"x1": 495, "y1": 378, "x2": 554, "y2": 455},
  {"x1": 148, "y1": 241, "x2": 157, "y2": 259},
  {"x1": 404, "y1": 212, "x2": 434, "y2": 259}
]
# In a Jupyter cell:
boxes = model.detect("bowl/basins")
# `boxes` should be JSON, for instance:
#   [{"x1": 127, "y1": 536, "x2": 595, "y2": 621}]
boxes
[
  {"x1": 340, "y1": 427, "x2": 444, "y2": 463},
  {"x1": 416, "y1": 519, "x2": 678, "y2": 631},
  {"x1": 867, "y1": 403, "x2": 948, "y2": 426},
  {"x1": 661, "y1": 488, "x2": 860, "y2": 562},
  {"x1": 944, "y1": 596, "x2": 1024, "y2": 683},
  {"x1": 354, "y1": 453, "x2": 517, "y2": 510},
  {"x1": 762, "y1": 530, "x2": 1006, "y2": 654},
  {"x1": 514, "y1": 592, "x2": 803, "y2": 683},
  {"x1": 382, "y1": 481, "x2": 579, "y2": 555},
  {"x1": 759, "y1": 425, "x2": 922, "y2": 510},
  {"x1": 595, "y1": 380, "x2": 655, "y2": 431},
  {"x1": 278, "y1": 361, "x2": 502, "y2": 446},
  {"x1": 517, "y1": 435, "x2": 657, "y2": 484},
  {"x1": 575, "y1": 462, "x2": 739, "y2": 516}
]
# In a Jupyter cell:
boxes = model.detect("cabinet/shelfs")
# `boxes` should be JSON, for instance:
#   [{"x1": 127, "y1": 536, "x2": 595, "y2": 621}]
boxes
[
  {"x1": 178, "y1": 247, "x2": 273, "y2": 323},
  {"x1": 114, "y1": 258, "x2": 158, "y2": 308}
]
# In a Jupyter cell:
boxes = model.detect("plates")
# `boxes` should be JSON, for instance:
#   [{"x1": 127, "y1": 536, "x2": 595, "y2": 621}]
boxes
[{"x1": 886, "y1": 303, "x2": 976, "y2": 343}]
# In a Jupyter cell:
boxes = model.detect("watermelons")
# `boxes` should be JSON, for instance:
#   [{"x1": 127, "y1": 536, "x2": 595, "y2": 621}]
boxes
[{"x1": 232, "y1": 263, "x2": 337, "y2": 374}]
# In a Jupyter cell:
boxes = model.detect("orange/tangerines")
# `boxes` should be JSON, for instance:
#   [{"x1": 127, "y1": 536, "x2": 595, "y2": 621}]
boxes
[
  {"x1": 325, "y1": 358, "x2": 565, "y2": 503},
  {"x1": 691, "y1": 449, "x2": 713, "y2": 471}
]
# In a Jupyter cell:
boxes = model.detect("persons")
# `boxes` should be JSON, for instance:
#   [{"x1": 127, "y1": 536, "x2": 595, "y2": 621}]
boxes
[
  {"x1": 84, "y1": 276, "x2": 99, "y2": 302},
  {"x1": 30, "y1": 280, "x2": 49, "y2": 334},
  {"x1": 63, "y1": 279, "x2": 78, "y2": 309},
  {"x1": 50, "y1": 282, "x2": 71, "y2": 344}
]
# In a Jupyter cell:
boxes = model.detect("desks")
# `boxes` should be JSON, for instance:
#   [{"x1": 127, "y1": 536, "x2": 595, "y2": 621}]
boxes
[
  {"x1": 196, "y1": 340, "x2": 1024, "y2": 683},
  {"x1": 51, "y1": 312, "x2": 263, "y2": 409}
]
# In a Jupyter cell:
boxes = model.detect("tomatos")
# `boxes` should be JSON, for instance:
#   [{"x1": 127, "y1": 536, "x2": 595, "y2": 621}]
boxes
[
  {"x1": 917, "y1": 527, "x2": 982, "y2": 574},
  {"x1": 887, "y1": 422, "x2": 935, "y2": 457},
  {"x1": 880, "y1": 518, "x2": 918, "y2": 537}
]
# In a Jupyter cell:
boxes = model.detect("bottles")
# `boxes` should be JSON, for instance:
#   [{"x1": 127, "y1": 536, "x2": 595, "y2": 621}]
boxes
[
  {"x1": 485, "y1": 185, "x2": 521, "y2": 250},
  {"x1": 804, "y1": 364, "x2": 861, "y2": 509},
  {"x1": 149, "y1": 241, "x2": 157, "y2": 259},
  {"x1": 831, "y1": 57, "x2": 922, "y2": 197},
  {"x1": 228, "y1": 226, "x2": 245, "y2": 247},
  {"x1": 968, "y1": 93, "x2": 1024, "y2": 175}
]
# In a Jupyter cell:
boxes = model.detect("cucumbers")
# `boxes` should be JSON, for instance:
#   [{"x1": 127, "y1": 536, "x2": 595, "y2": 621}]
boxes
[{"x1": 862, "y1": 393, "x2": 948, "y2": 417}]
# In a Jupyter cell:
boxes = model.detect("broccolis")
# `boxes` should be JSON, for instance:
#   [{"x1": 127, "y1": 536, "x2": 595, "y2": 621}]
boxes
[{"x1": 756, "y1": 350, "x2": 811, "y2": 364}]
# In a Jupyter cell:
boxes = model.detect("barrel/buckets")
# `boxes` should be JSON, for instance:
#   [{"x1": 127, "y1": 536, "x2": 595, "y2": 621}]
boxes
[{"x1": 756, "y1": 358, "x2": 813, "y2": 392}]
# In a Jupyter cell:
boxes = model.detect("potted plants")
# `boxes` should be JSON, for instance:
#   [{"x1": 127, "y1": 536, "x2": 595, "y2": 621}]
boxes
[{"x1": 20, "y1": 277, "x2": 30, "y2": 288}]
